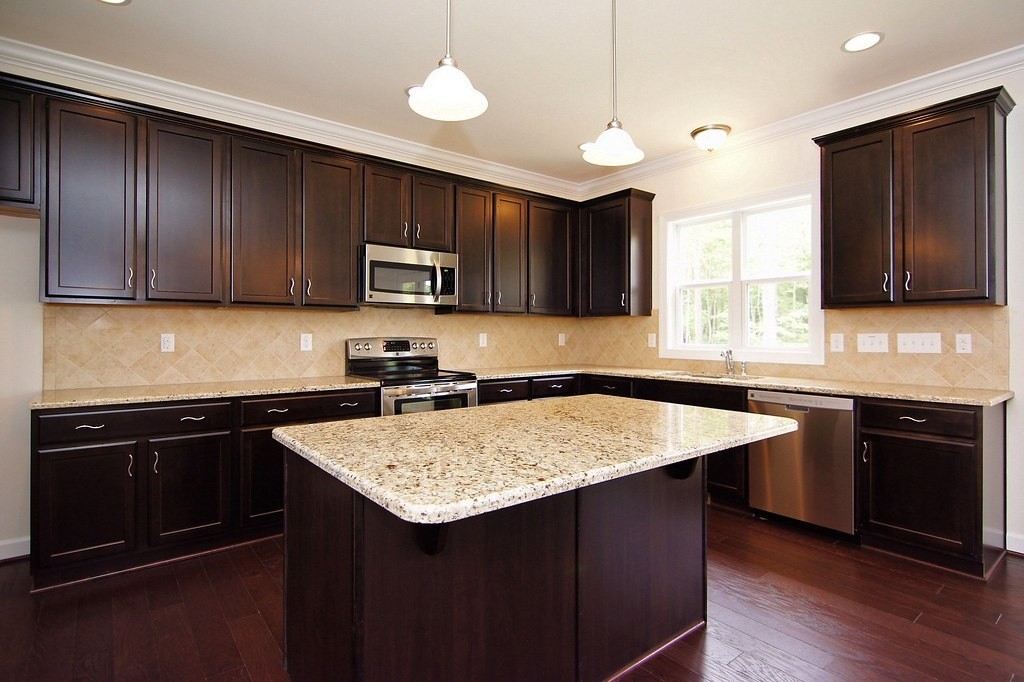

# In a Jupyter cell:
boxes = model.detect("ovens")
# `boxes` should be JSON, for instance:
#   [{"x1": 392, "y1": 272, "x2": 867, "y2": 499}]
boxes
[{"x1": 380, "y1": 380, "x2": 479, "y2": 418}]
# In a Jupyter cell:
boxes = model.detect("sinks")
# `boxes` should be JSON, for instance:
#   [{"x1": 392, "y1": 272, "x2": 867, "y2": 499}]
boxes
[{"x1": 662, "y1": 370, "x2": 765, "y2": 381}]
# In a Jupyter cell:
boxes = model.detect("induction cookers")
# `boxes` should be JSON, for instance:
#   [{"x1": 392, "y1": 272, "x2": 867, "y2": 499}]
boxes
[{"x1": 345, "y1": 336, "x2": 476, "y2": 385}]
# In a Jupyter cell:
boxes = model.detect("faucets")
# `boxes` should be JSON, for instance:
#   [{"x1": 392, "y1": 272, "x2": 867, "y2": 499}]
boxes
[{"x1": 720, "y1": 349, "x2": 734, "y2": 374}]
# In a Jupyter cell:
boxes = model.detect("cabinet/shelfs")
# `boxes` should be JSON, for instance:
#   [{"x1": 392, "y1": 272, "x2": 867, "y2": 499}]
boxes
[
  {"x1": 0, "y1": 71, "x2": 653, "y2": 318},
  {"x1": 854, "y1": 397, "x2": 1008, "y2": 579},
  {"x1": 278, "y1": 446, "x2": 707, "y2": 682},
  {"x1": 29, "y1": 387, "x2": 382, "y2": 588},
  {"x1": 478, "y1": 376, "x2": 748, "y2": 501},
  {"x1": 811, "y1": 85, "x2": 1017, "y2": 309}
]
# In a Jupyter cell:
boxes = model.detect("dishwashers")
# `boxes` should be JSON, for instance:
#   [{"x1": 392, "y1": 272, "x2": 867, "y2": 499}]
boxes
[{"x1": 747, "y1": 389, "x2": 855, "y2": 536}]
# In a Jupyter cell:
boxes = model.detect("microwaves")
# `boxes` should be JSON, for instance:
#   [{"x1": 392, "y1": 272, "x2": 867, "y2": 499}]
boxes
[{"x1": 361, "y1": 243, "x2": 458, "y2": 310}]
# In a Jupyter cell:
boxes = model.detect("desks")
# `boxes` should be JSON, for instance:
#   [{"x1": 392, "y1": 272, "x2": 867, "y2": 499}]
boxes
[{"x1": 271, "y1": 393, "x2": 799, "y2": 524}]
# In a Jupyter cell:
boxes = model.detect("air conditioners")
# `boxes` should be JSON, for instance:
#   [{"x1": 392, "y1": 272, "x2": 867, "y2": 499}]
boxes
[{"x1": 692, "y1": 125, "x2": 732, "y2": 160}]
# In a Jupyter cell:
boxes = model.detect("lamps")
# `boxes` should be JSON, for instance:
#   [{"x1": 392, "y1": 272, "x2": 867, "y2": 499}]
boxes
[
  {"x1": 840, "y1": 31, "x2": 884, "y2": 54},
  {"x1": 578, "y1": 1, "x2": 645, "y2": 167},
  {"x1": 404, "y1": 0, "x2": 489, "y2": 122}
]
